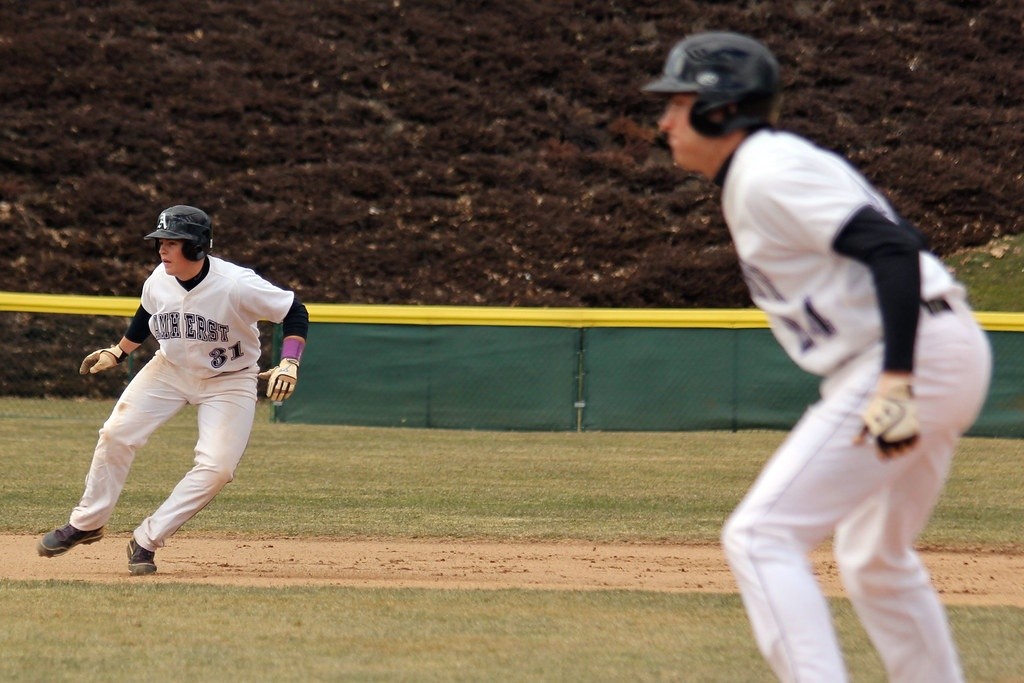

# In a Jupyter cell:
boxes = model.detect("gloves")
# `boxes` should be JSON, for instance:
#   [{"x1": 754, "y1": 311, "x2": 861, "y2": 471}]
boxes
[
  {"x1": 258, "y1": 358, "x2": 306, "y2": 402},
  {"x1": 852, "y1": 371, "x2": 924, "y2": 464},
  {"x1": 78, "y1": 335, "x2": 141, "y2": 376}
]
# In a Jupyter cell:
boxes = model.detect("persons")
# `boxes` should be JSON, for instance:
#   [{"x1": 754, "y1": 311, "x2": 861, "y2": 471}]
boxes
[
  {"x1": 37, "y1": 206, "x2": 309, "y2": 574},
  {"x1": 641, "y1": 33, "x2": 993, "y2": 683}
]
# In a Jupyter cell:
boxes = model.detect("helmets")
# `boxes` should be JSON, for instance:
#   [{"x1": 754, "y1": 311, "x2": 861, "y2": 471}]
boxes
[
  {"x1": 641, "y1": 32, "x2": 787, "y2": 139},
  {"x1": 143, "y1": 205, "x2": 213, "y2": 261}
]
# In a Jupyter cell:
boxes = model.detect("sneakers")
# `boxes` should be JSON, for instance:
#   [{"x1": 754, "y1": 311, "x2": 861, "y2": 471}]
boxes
[
  {"x1": 36, "y1": 521, "x2": 104, "y2": 559},
  {"x1": 126, "y1": 537, "x2": 158, "y2": 576}
]
